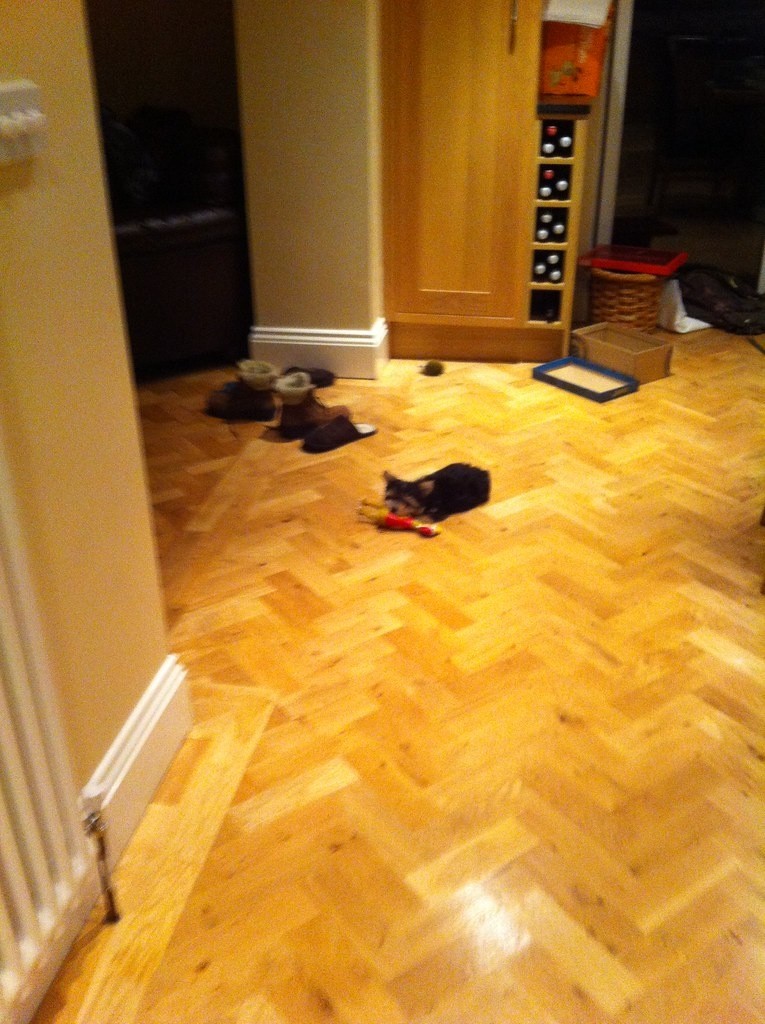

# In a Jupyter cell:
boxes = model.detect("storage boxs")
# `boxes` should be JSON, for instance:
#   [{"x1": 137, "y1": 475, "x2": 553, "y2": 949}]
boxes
[
  {"x1": 588, "y1": 266, "x2": 664, "y2": 332},
  {"x1": 571, "y1": 321, "x2": 674, "y2": 385},
  {"x1": 542, "y1": 18, "x2": 611, "y2": 96}
]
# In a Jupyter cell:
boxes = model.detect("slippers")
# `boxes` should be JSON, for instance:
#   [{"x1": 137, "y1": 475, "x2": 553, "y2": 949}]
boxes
[{"x1": 304, "y1": 415, "x2": 376, "y2": 451}]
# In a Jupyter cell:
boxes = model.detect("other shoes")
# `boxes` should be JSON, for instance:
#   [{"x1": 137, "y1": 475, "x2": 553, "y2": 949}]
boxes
[{"x1": 284, "y1": 366, "x2": 336, "y2": 387}]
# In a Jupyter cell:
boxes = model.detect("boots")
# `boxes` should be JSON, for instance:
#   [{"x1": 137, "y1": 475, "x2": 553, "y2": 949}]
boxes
[{"x1": 206, "y1": 361, "x2": 351, "y2": 436}]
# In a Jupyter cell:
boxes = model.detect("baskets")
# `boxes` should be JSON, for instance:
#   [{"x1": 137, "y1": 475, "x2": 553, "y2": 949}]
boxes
[{"x1": 588, "y1": 269, "x2": 663, "y2": 334}]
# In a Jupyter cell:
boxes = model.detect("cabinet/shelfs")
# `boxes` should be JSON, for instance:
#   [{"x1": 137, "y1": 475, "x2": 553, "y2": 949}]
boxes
[{"x1": 377, "y1": 0, "x2": 616, "y2": 362}]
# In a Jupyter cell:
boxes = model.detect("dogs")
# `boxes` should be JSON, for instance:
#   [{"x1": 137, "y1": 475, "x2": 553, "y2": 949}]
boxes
[{"x1": 382, "y1": 462, "x2": 491, "y2": 523}]
